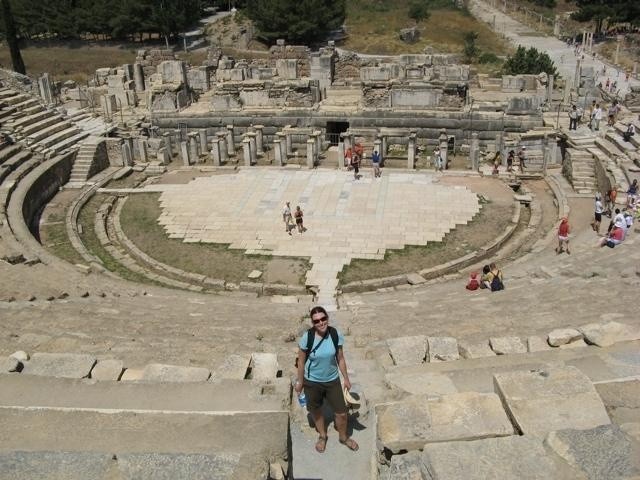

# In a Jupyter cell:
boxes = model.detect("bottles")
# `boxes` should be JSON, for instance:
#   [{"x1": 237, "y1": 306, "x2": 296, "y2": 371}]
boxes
[{"x1": 297, "y1": 393, "x2": 307, "y2": 407}]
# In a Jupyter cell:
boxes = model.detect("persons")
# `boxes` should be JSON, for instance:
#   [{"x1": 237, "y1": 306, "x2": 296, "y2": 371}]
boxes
[
  {"x1": 433, "y1": 146, "x2": 443, "y2": 171},
  {"x1": 568, "y1": 100, "x2": 621, "y2": 131},
  {"x1": 565, "y1": 40, "x2": 631, "y2": 92},
  {"x1": 623, "y1": 122, "x2": 635, "y2": 142},
  {"x1": 593, "y1": 179, "x2": 639, "y2": 248},
  {"x1": 492, "y1": 146, "x2": 529, "y2": 175},
  {"x1": 283, "y1": 201, "x2": 304, "y2": 236},
  {"x1": 295, "y1": 306, "x2": 359, "y2": 453},
  {"x1": 345, "y1": 148, "x2": 363, "y2": 179},
  {"x1": 371, "y1": 150, "x2": 381, "y2": 178},
  {"x1": 556, "y1": 218, "x2": 573, "y2": 255},
  {"x1": 466, "y1": 264, "x2": 504, "y2": 291}
]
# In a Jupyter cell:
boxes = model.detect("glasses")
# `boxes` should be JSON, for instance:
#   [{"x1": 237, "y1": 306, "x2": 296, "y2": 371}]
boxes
[{"x1": 314, "y1": 317, "x2": 326, "y2": 323}]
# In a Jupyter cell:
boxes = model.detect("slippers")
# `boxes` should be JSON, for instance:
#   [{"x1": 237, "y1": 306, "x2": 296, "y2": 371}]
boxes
[
  {"x1": 339, "y1": 436, "x2": 359, "y2": 451},
  {"x1": 316, "y1": 436, "x2": 328, "y2": 453}
]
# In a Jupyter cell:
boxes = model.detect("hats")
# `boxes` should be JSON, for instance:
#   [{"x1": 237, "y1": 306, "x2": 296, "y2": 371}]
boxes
[
  {"x1": 614, "y1": 221, "x2": 621, "y2": 228},
  {"x1": 343, "y1": 387, "x2": 361, "y2": 408}
]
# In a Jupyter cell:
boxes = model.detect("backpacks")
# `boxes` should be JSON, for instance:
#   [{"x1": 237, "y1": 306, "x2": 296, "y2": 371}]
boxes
[{"x1": 490, "y1": 270, "x2": 504, "y2": 291}]
